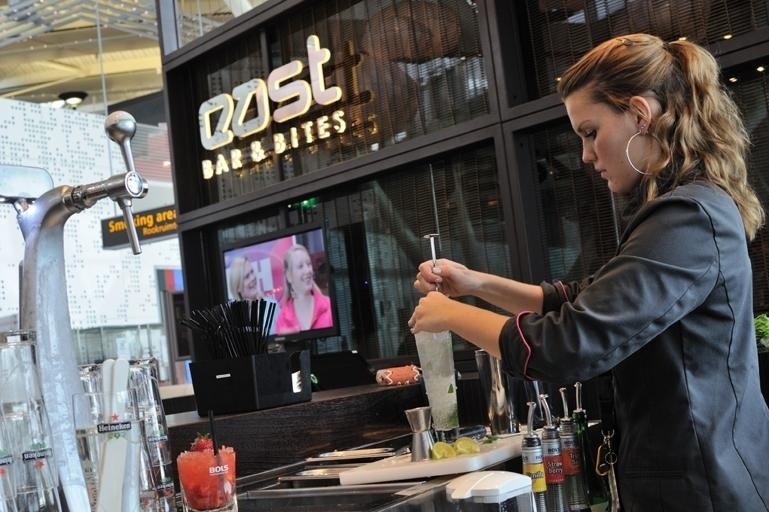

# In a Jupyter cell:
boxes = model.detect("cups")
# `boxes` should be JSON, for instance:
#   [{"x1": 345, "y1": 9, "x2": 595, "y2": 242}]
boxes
[
  {"x1": 0, "y1": 339, "x2": 177, "y2": 512},
  {"x1": 475, "y1": 347, "x2": 548, "y2": 436},
  {"x1": 412, "y1": 331, "x2": 460, "y2": 444},
  {"x1": 177, "y1": 447, "x2": 239, "y2": 512}
]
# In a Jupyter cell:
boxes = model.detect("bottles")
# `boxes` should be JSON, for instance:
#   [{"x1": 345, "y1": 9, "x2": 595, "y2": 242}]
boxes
[
  {"x1": 404, "y1": 405, "x2": 435, "y2": 463},
  {"x1": 519, "y1": 408, "x2": 612, "y2": 511}
]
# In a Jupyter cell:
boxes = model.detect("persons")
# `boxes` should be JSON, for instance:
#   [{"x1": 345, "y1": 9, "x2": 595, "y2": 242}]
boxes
[
  {"x1": 403, "y1": 32, "x2": 769, "y2": 512},
  {"x1": 229, "y1": 255, "x2": 281, "y2": 337},
  {"x1": 271, "y1": 243, "x2": 334, "y2": 334}
]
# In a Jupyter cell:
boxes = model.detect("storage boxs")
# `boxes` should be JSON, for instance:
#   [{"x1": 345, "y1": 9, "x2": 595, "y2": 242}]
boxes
[{"x1": 184, "y1": 347, "x2": 314, "y2": 419}]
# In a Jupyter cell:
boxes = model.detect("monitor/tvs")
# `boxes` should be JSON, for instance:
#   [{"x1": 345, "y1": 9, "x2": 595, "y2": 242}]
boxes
[{"x1": 216, "y1": 217, "x2": 342, "y2": 344}]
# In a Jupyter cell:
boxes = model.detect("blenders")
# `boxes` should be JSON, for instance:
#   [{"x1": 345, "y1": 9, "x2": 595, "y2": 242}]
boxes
[{"x1": 445, "y1": 470, "x2": 537, "y2": 512}]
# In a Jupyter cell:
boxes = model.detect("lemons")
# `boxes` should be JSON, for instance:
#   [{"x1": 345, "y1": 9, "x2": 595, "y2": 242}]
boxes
[
  {"x1": 454, "y1": 437, "x2": 480, "y2": 454},
  {"x1": 431, "y1": 442, "x2": 456, "y2": 460}
]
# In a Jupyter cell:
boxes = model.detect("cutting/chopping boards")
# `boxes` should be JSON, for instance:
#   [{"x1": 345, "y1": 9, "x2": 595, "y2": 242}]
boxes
[{"x1": 338, "y1": 424, "x2": 542, "y2": 487}]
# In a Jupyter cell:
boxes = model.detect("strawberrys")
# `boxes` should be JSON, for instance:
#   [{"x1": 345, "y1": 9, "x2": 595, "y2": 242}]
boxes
[{"x1": 190, "y1": 432, "x2": 213, "y2": 451}]
